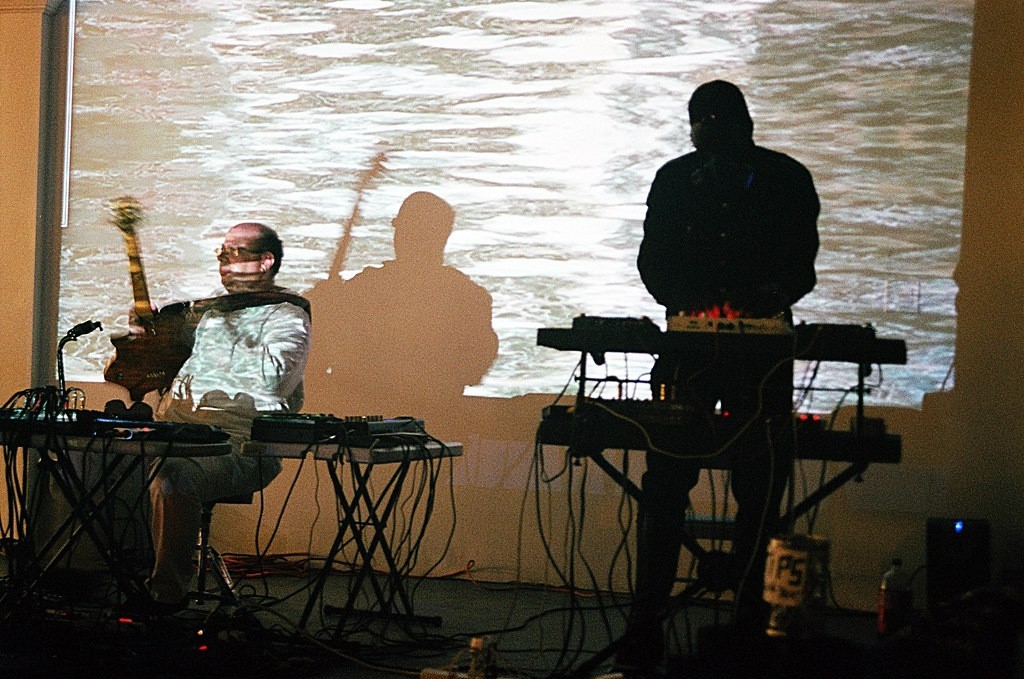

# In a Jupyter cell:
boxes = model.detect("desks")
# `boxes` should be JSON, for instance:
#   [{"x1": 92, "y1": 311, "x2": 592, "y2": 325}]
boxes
[
  {"x1": 0, "y1": 430, "x2": 232, "y2": 625},
  {"x1": 240, "y1": 440, "x2": 464, "y2": 650}
]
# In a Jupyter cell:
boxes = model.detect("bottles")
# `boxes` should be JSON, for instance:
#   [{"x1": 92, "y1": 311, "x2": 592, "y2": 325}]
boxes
[{"x1": 876, "y1": 558, "x2": 914, "y2": 637}]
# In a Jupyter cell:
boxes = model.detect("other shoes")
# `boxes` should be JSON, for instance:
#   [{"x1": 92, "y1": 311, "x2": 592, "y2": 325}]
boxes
[
  {"x1": 127, "y1": 590, "x2": 188, "y2": 621},
  {"x1": 613, "y1": 614, "x2": 666, "y2": 677}
]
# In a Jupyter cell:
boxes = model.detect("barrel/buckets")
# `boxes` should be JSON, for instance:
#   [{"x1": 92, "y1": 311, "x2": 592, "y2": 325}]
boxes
[{"x1": 759, "y1": 532, "x2": 832, "y2": 643}]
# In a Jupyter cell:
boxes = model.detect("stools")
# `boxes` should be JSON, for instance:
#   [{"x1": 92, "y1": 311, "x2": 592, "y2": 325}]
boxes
[
  {"x1": 195, "y1": 494, "x2": 253, "y2": 607},
  {"x1": 684, "y1": 514, "x2": 735, "y2": 654}
]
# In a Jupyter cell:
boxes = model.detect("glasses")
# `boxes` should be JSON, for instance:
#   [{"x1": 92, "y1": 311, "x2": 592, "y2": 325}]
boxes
[{"x1": 214, "y1": 246, "x2": 266, "y2": 259}]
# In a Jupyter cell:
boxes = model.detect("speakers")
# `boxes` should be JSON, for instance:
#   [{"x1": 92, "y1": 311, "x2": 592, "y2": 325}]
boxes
[{"x1": 925, "y1": 516, "x2": 989, "y2": 623}]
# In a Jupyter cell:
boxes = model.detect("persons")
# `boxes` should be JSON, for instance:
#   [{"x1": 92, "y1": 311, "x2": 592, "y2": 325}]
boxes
[
  {"x1": 33, "y1": 223, "x2": 312, "y2": 619},
  {"x1": 612, "y1": 80, "x2": 821, "y2": 679}
]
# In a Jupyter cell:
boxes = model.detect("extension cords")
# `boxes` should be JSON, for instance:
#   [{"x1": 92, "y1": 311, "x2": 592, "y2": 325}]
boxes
[{"x1": 420, "y1": 666, "x2": 485, "y2": 679}]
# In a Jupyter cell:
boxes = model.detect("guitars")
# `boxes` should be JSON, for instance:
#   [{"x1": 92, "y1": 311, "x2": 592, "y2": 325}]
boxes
[{"x1": 102, "y1": 195, "x2": 191, "y2": 391}]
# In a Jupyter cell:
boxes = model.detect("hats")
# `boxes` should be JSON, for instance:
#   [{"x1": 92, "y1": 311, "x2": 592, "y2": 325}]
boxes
[{"x1": 687, "y1": 78, "x2": 753, "y2": 126}]
